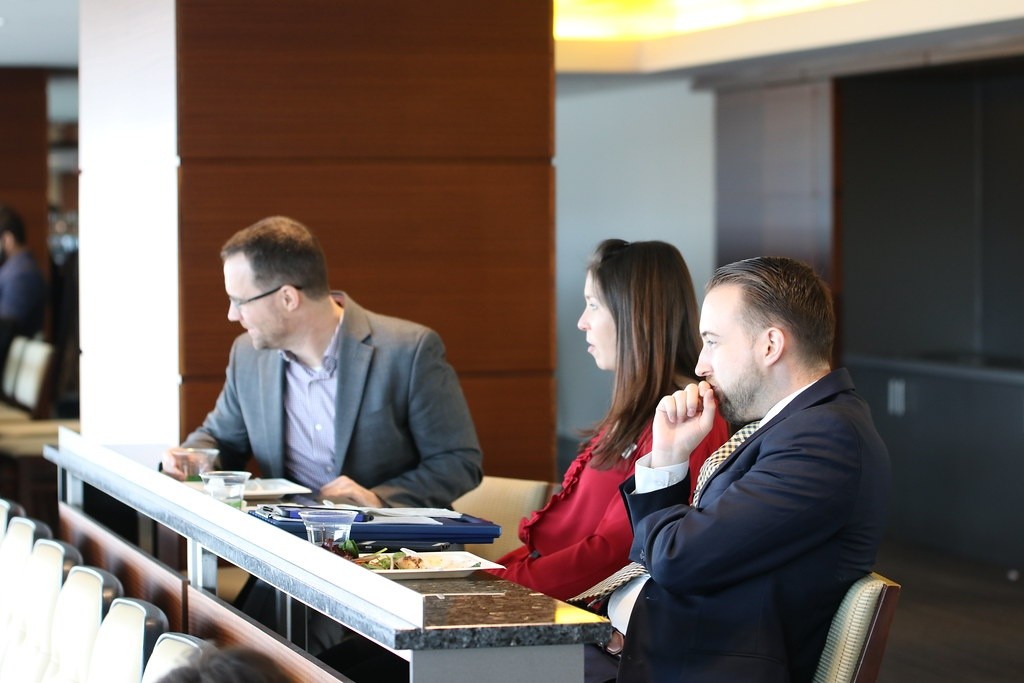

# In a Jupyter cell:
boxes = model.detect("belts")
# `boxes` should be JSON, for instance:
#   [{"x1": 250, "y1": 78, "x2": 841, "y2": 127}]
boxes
[{"x1": 594, "y1": 628, "x2": 626, "y2": 658}]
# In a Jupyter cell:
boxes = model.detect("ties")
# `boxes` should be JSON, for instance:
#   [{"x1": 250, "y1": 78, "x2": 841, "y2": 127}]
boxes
[{"x1": 566, "y1": 423, "x2": 760, "y2": 601}]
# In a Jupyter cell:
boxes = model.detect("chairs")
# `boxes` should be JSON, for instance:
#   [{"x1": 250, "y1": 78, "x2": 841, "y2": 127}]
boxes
[
  {"x1": 1, "y1": 496, "x2": 281, "y2": 683},
  {"x1": 813, "y1": 573, "x2": 901, "y2": 682},
  {"x1": 449, "y1": 475, "x2": 562, "y2": 580},
  {"x1": 0, "y1": 336, "x2": 61, "y2": 456}
]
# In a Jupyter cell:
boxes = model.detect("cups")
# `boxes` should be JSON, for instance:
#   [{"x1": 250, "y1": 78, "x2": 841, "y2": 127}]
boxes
[
  {"x1": 168, "y1": 449, "x2": 220, "y2": 478},
  {"x1": 298, "y1": 510, "x2": 358, "y2": 547},
  {"x1": 199, "y1": 470, "x2": 251, "y2": 509}
]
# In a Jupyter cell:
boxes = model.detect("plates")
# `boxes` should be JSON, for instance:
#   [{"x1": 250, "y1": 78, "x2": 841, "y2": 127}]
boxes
[
  {"x1": 359, "y1": 549, "x2": 506, "y2": 580},
  {"x1": 244, "y1": 477, "x2": 312, "y2": 499}
]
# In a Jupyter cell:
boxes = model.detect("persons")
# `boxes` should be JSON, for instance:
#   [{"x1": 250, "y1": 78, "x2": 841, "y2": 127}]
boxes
[
  {"x1": 160, "y1": 214, "x2": 488, "y2": 656},
  {"x1": 1, "y1": 199, "x2": 47, "y2": 352},
  {"x1": 314, "y1": 241, "x2": 894, "y2": 683}
]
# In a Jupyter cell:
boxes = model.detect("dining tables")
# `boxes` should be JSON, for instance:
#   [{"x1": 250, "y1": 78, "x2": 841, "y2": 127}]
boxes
[{"x1": 39, "y1": 425, "x2": 617, "y2": 683}]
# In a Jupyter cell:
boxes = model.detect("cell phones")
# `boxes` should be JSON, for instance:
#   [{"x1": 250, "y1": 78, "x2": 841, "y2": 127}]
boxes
[{"x1": 276, "y1": 505, "x2": 368, "y2": 523}]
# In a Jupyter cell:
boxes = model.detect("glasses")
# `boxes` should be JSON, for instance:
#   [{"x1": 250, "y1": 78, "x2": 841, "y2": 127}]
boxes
[{"x1": 227, "y1": 284, "x2": 301, "y2": 305}]
